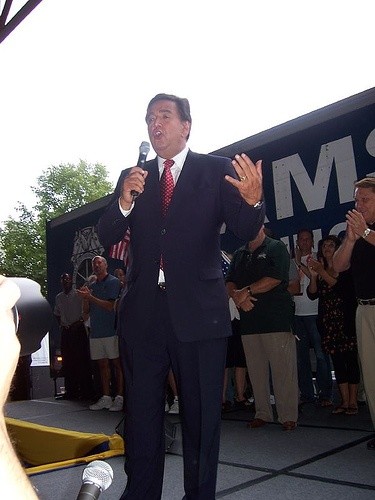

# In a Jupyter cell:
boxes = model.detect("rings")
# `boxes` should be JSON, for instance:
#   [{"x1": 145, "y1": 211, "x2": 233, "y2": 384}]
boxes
[{"x1": 240, "y1": 176, "x2": 246, "y2": 180}]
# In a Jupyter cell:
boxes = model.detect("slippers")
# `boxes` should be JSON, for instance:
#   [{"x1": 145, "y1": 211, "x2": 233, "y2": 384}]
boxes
[
  {"x1": 221, "y1": 403, "x2": 226, "y2": 410},
  {"x1": 345, "y1": 407, "x2": 359, "y2": 414},
  {"x1": 235, "y1": 397, "x2": 254, "y2": 407},
  {"x1": 331, "y1": 406, "x2": 348, "y2": 414}
]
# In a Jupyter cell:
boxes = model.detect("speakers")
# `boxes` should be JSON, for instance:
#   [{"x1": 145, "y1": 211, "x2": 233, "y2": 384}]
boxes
[{"x1": 115, "y1": 415, "x2": 176, "y2": 452}]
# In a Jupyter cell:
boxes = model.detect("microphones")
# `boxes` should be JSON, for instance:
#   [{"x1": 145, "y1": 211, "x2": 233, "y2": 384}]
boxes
[
  {"x1": 130, "y1": 142, "x2": 150, "y2": 196},
  {"x1": 76, "y1": 460, "x2": 113, "y2": 500}
]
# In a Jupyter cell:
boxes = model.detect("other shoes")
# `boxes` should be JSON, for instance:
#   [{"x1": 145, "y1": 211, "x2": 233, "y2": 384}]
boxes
[
  {"x1": 282, "y1": 421, "x2": 297, "y2": 431},
  {"x1": 303, "y1": 402, "x2": 314, "y2": 408},
  {"x1": 55, "y1": 394, "x2": 71, "y2": 400},
  {"x1": 321, "y1": 399, "x2": 333, "y2": 407},
  {"x1": 367, "y1": 439, "x2": 375, "y2": 449},
  {"x1": 246, "y1": 419, "x2": 264, "y2": 427},
  {"x1": 80, "y1": 395, "x2": 89, "y2": 400}
]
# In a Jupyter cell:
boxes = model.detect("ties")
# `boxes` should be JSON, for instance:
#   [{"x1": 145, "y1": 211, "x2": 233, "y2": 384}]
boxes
[{"x1": 159, "y1": 159, "x2": 175, "y2": 273}]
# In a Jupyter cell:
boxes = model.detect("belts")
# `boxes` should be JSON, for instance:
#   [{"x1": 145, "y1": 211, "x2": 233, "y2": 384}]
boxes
[
  {"x1": 355, "y1": 297, "x2": 375, "y2": 305},
  {"x1": 157, "y1": 285, "x2": 168, "y2": 296}
]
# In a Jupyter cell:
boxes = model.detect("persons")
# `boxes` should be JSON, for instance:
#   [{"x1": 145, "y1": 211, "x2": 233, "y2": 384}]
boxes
[
  {"x1": 288, "y1": 229, "x2": 335, "y2": 406},
  {"x1": 77, "y1": 255, "x2": 123, "y2": 411},
  {"x1": 305, "y1": 235, "x2": 360, "y2": 415},
  {"x1": 220, "y1": 297, "x2": 254, "y2": 413},
  {"x1": 0, "y1": 274, "x2": 39, "y2": 500},
  {"x1": 98, "y1": 93, "x2": 266, "y2": 500},
  {"x1": 332, "y1": 178, "x2": 375, "y2": 451},
  {"x1": 53, "y1": 272, "x2": 93, "y2": 404},
  {"x1": 225, "y1": 224, "x2": 298, "y2": 429},
  {"x1": 164, "y1": 367, "x2": 179, "y2": 414}
]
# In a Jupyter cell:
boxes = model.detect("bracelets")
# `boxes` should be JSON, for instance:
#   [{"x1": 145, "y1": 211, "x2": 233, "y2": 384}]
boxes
[
  {"x1": 247, "y1": 286, "x2": 252, "y2": 295},
  {"x1": 362, "y1": 228, "x2": 370, "y2": 238}
]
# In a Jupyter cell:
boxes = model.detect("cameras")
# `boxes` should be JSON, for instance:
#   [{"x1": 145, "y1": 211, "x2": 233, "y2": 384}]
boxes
[{"x1": 4, "y1": 274, "x2": 53, "y2": 356}]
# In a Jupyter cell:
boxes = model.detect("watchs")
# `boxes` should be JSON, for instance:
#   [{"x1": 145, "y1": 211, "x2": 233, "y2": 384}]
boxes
[{"x1": 254, "y1": 200, "x2": 264, "y2": 209}]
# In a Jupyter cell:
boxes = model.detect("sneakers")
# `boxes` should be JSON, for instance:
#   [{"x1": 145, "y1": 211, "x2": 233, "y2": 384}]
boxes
[
  {"x1": 164, "y1": 399, "x2": 169, "y2": 412},
  {"x1": 88, "y1": 398, "x2": 113, "y2": 410},
  {"x1": 168, "y1": 399, "x2": 179, "y2": 414},
  {"x1": 109, "y1": 397, "x2": 123, "y2": 411}
]
